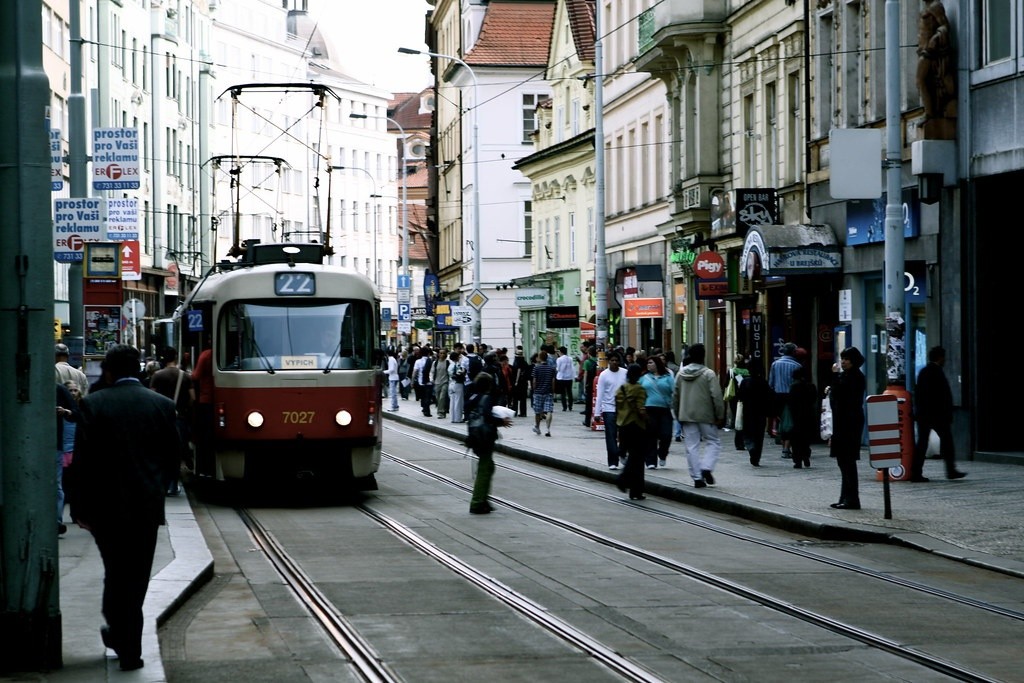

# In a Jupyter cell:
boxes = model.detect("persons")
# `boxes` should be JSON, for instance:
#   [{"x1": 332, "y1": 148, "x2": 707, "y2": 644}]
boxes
[
  {"x1": 88, "y1": 332, "x2": 236, "y2": 497},
  {"x1": 911, "y1": 346, "x2": 967, "y2": 482},
  {"x1": 825, "y1": 347, "x2": 865, "y2": 509},
  {"x1": 722, "y1": 343, "x2": 819, "y2": 469},
  {"x1": 51, "y1": 343, "x2": 88, "y2": 534},
  {"x1": 673, "y1": 344, "x2": 724, "y2": 488},
  {"x1": 381, "y1": 341, "x2": 684, "y2": 442},
  {"x1": 916, "y1": 2, "x2": 951, "y2": 128},
  {"x1": 309, "y1": 325, "x2": 356, "y2": 357},
  {"x1": 71, "y1": 346, "x2": 183, "y2": 671},
  {"x1": 615, "y1": 365, "x2": 649, "y2": 498},
  {"x1": 595, "y1": 352, "x2": 628, "y2": 470},
  {"x1": 466, "y1": 373, "x2": 514, "y2": 513},
  {"x1": 637, "y1": 356, "x2": 675, "y2": 468}
]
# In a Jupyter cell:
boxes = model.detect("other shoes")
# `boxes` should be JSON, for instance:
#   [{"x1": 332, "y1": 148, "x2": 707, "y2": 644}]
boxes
[
  {"x1": 470, "y1": 503, "x2": 497, "y2": 514},
  {"x1": 120, "y1": 659, "x2": 143, "y2": 669},
  {"x1": 101, "y1": 628, "x2": 118, "y2": 654},
  {"x1": 609, "y1": 465, "x2": 617, "y2": 469},
  {"x1": 620, "y1": 457, "x2": 627, "y2": 465},
  {"x1": 695, "y1": 471, "x2": 715, "y2": 488},
  {"x1": 802, "y1": 457, "x2": 810, "y2": 467},
  {"x1": 947, "y1": 470, "x2": 965, "y2": 479},
  {"x1": 676, "y1": 437, "x2": 681, "y2": 442},
  {"x1": 58, "y1": 525, "x2": 66, "y2": 534},
  {"x1": 910, "y1": 476, "x2": 928, "y2": 482},
  {"x1": 647, "y1": 465, "x2": 655, "y2": 469},
  {"x1": 532, "y1": 428, "x2": 540, "y2": 434},
  {"x1": 793, "y1": 464, "x2": 802, "y2": 468},
  {"x1": 781, "y1": 451, "x2": 791, "y2": 458},
  {"x1": 630, "y1": 494, "x2": 647, "y2": 501},
  {"x1": 546, "y1": 433, "x2": 550, "y2": 436},
  {"x1": 660, "y1": 459, "x2": 665, "y2": 466}
]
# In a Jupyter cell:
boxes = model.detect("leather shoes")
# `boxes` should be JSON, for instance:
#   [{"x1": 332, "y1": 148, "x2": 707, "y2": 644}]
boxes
[{"x1": 831, "y1": 499, "x2": 860, "y2": 509}]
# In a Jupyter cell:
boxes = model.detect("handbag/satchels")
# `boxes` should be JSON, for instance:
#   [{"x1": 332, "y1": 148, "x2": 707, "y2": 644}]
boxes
[
  {"x1": 723, "y1": 369, "x2": 735, "y2": 401},
  {"x1": 451, "y1": 365, "x2": 467, "y2": 384},
  {"x1": 735, "y1": 400, "x2": 743, "y2": 430}
]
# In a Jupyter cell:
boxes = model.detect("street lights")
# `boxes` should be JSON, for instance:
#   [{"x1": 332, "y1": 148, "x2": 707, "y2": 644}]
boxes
[
  {"x1": 329, "y1": 166, "x2": 383, "y2": 312},
  {"x1": 350, "y1": 113, "x2": 408, "y2": 344},
  {"x1": 400, "y1": 46, "x2": 484, "y2": 340},
  {"x1": 149, "y1": 32, "x2": 180, "y2": 314}
]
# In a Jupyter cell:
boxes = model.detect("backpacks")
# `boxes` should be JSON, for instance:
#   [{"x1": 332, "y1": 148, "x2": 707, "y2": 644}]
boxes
[
  {"x1": 468, "y1": 394, "x2": 492, "y2": 445},
  {"x1": 466, "y1": 356, "x2": 482, "y2": 372}
]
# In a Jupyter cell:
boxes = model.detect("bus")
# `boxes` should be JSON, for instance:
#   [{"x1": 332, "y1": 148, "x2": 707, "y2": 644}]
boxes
[{"x1": 148, "y1": 244, "x2": 384, "y2": 505}]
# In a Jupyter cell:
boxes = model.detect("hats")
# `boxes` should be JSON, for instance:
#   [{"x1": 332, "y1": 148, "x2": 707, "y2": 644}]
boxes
[
  {"x1": 840, "y1": 347, "x2": 865, "y2": 368},
  {"x1": 515, "y1": 350, "x2": 523, "y2": 357},
  {"x1": 55, "y1": 343, "x2": 69, "y2": 355}
]
